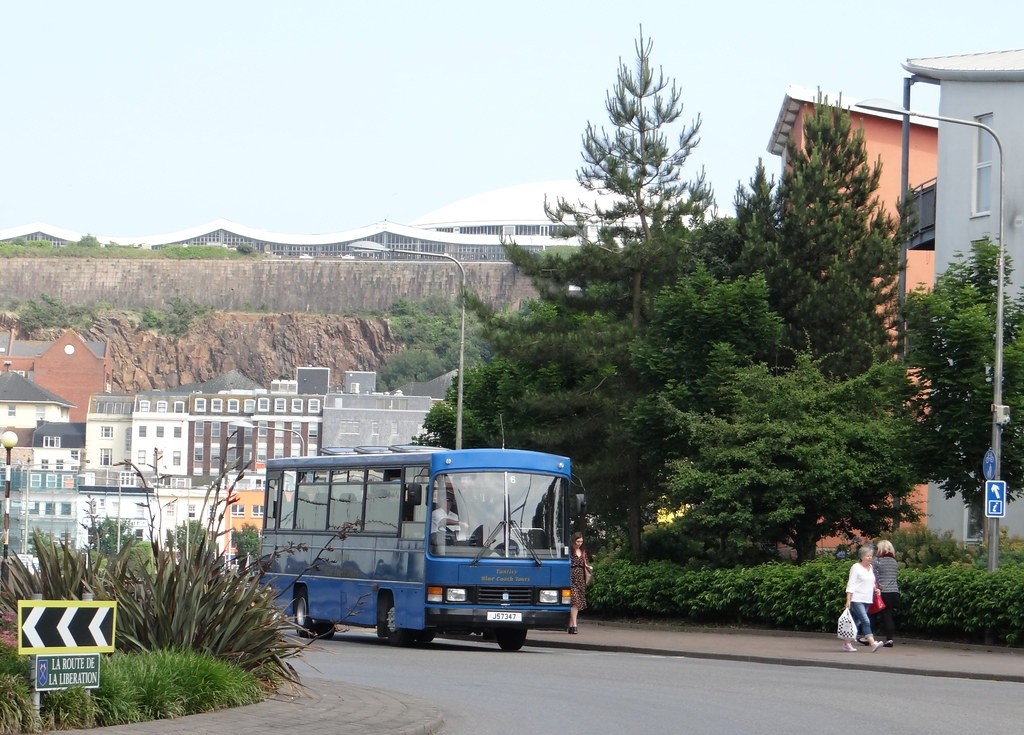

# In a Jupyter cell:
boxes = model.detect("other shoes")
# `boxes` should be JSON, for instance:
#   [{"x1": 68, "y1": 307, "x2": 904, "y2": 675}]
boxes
[
  {"x1": 568, "y1": 625, "x2": 577, "y2": 634},
  {"x1": 856, "y1": 638, "x2": 869, "y2": 646},
  {"x1": 882, "y1": 642, "x2": 894, "y2": 647}
]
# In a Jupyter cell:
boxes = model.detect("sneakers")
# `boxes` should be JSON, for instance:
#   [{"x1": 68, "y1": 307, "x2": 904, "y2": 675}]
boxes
[
  {"x1": 843, "y1": 643, "x2": 857, "y2": 652},
  {"x1": 871, "y1": 641, "x2": 883, "y2": 653}
]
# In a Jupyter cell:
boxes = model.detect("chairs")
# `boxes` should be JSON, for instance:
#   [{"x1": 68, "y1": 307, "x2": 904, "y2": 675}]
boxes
[{"x1": 266, "y1": 487, "x2": 549, "y2": 549}]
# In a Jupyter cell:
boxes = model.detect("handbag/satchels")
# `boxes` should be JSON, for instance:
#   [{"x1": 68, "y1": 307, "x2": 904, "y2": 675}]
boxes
[
  {"x1": 583, "y1": 550, "x2": 593, "y2": 585},
  {"x1": 867, "y1": 587, "x2": 886, "y2": 614},
  {"x1": 837, "y1": 607, "x2": 858, "y2": 641}
]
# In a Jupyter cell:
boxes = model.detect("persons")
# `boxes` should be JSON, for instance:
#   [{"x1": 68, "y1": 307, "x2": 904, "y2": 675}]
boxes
[
  {"x1": 857, "y1": 540, "x2": 900, "y2": 647},
  {"x1": 841, "y1": 546, "x2": 883, "y2": 653},
  {"x1": 568, "y1": 532, "x2": 593, "y2": 634},
  {"x1": 431, "y1": 491, "x2": 469, "y2": 533}
]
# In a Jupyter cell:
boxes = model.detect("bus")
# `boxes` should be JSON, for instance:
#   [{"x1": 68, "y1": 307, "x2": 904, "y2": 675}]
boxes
[{"x1": 257, "y1": 448, "x2": 576, "y2": 655}]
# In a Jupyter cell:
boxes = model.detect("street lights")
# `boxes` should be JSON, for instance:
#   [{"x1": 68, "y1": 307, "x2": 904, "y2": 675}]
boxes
[
  {"x1": 856, "y1": 98, "x2": 1008, "y2": 577},
  {"x1": 346, "y1": 237, "x2": 467, "y2": 455}
]
